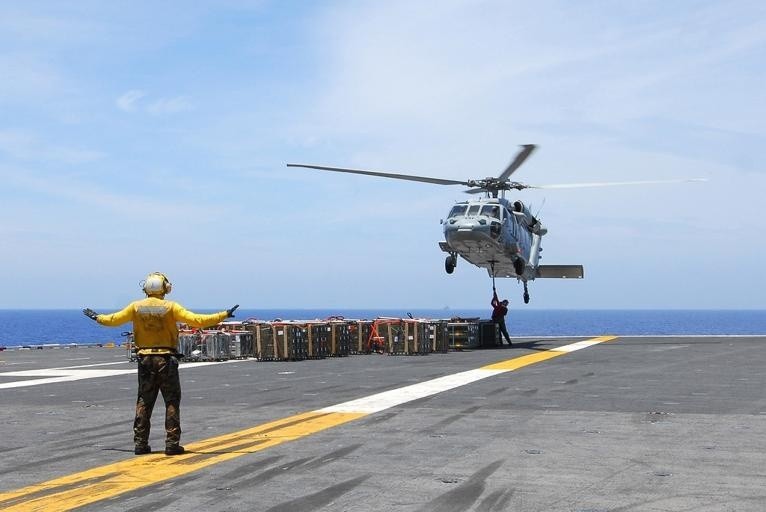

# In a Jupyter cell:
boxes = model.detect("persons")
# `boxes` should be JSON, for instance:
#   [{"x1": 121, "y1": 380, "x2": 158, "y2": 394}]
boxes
[
  {"x1": 80, "y1": 270, "x2": 241, "y2": 455},
  {"x1": 490, "y1": 287, "x2": 513, "y2": 347}
]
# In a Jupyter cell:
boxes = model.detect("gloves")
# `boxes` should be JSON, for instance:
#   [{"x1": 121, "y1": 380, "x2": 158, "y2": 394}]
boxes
[
  {"x1": 227, "y1": 305, "x2": 239, "y2": 318},
  {"x1": 83, "y1": 308, "x2": 99, "y2": 320}
]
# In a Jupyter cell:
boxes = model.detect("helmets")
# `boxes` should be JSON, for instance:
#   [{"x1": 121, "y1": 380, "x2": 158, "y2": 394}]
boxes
[{"x1": 138, "y1": 272, "x2": 172, "y2": 298}]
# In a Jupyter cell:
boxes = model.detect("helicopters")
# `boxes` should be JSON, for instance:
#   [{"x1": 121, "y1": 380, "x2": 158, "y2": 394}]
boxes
[{"x1": 286, "y1": 143, "x2": 708, "y2": 303}]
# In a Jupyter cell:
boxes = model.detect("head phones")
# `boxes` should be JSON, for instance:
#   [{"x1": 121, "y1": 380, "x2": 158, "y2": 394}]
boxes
[{"x1": 143, "y1": 271, "x2": 172, "y2": 294}]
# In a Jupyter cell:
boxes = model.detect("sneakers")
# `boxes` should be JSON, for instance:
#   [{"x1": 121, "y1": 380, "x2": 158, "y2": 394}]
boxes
[
  {"x1": 165, "y1": 446, "x2": 184, "y2": 455},
  {"x1": 135, "y1": 445, "x2": 151, "y2": 455}
]
